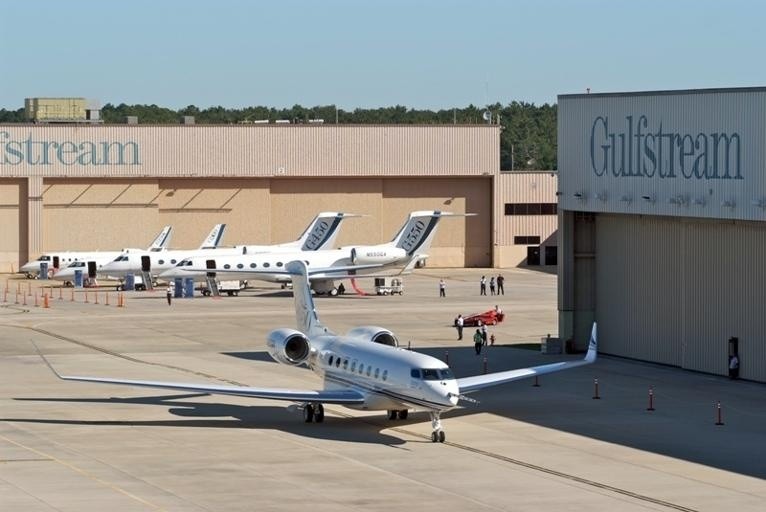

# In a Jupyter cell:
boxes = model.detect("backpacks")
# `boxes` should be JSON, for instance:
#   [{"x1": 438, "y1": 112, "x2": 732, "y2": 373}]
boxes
[{"x1": 476, "y1": 335, "x2": 481, "y2": 343}]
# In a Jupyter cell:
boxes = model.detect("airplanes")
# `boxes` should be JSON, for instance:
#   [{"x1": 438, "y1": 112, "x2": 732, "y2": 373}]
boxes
[
  {"x1": 18, "y1": 225, "x2": 175, "y2": 281},
  {"x1": 150, "y1": 209, "x2": 479, "y2": 297},
  {"x1": 30, "y1": 261, "x2": 598, "y2": 443},
  {"x1": 94, "y1": 212, "x2": 371, "y2": 293},
  {"x1": 52, "y1": 222, "x2": 228, "y2": 288}
]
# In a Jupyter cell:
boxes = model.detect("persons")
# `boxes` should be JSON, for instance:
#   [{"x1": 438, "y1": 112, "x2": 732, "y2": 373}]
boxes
[
  {"x1": 482, "y1": 322, "x2": 487, "y2": 346},
  {"x1": 440, "y1": 280, "x2": 446, "y2": 297},
  {"x1": 474, "y1": 329, "x2": 482, "y2": 355},
  {"x1": 456, "y1": 314, "x2": 464, "y2": 340},
  {"x1": 339, "y1": 283, "x2": 345, "y2": 294},
  {"x1": 167, "y1": 284, "x2": 172, "y2": 306},
  {"x1": 497, "y1": 273, "x2": 504, "y2": 295},
  {"x1": 729, "y1": 352, "x2": 739, "y2": 380},
  {"x1": 480, "y1": 276, "x2": 486, "y2": 295},
  {"x1": 490, "y1": 277, "x2": 495, "y2": 296}
]
